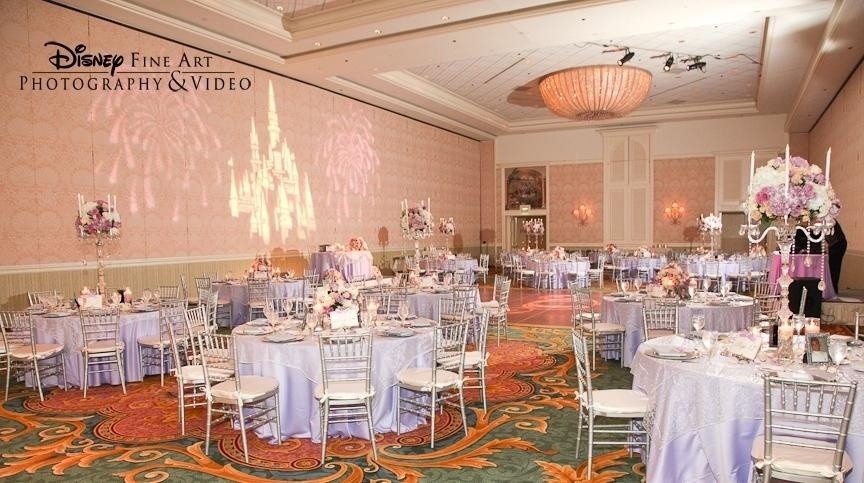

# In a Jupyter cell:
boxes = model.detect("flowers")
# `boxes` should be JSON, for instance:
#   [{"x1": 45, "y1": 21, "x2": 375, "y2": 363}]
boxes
[
  {"x1": 314, "y1": 268, "x2": 363, "y2": 317},
  {"x1": 438, "y1": 220, "x2": 456, "y2": 235},
  {"x1": 602, "y1": 244, "x2": 617, "y2": 253},
  {"x1": 73, "y1": 200, "x2": 120, "y2": 237},
  {"x1": 399, "y1": 206, "x2": 434, "y2": 240},
  {"x1": 743, "y1": 156, "x2": 841, "y2": 237},
  {"x1": 349, "y1": 236, "x2": 365, "y2": 252},
  {"x1": 650, "y1": 261, "x2": 691, "y2": 298},
  {"x1": 246, "y1": 257, "x2": 272, "y2": 278},
  {"x1": 698, "y1": 216, "x2": 722, "y2": 233},
  {"x1": 523, "y1": 217, "x2": 544, "y2": 235}
]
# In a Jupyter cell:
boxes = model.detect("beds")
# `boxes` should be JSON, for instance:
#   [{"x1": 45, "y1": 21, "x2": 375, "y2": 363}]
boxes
[{"x1": 12, "y1": 303, "x2": 188, "y2": 390}]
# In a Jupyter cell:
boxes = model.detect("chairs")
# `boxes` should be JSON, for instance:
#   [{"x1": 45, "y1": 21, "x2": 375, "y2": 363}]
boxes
[
  {"x1": 752, "y1": 258, "x2": 766, "y2": 281},
  {"x1": 163, "y1": 316, "x2": 235, "y2": 435},
  {"x1": 28, "y1": 291, "x2": 56, "y2": 306},
  {"x1": 750, "y1": 372, "x2": 858, "y2": 483},
  {"x1": 425, "y1": 253, "x2": 445, "y2": 282},
  {"x1": 315, "y1": 334, "x2": 378, "y2": 464},
  {"x1": 753, "y1": 296, "x2": 784, "y2": 328},
  {"x1": 617, "y1": 279, "x2": 635, "y2": 291},
  {"x1": 567, "y1": 258, "x2": 588, "y2": 289},
  {"x1": 512, "y1": 257, "x2": 536, "y2": 288},
  {"x1": 406, "y1": 256, "x2": 425, "y2": 272},
  {"x1": 180, "y1": 274, "x2": 199, "y2": 304},
  {"x1": 0, "y1": 310, "x2": 67, "y2": 402},
  {"x1": 500, "y1": 252, "x2": 512, "y2": 280},
  {"x1": 754, "y1": 280, "x2": 778, "y2": 316},
  {"x1": 576, "y1": 289, "x2": 626, "y2": 370},
  {"x1": 243, "y1": 276, "x2": 269, "y2": 321},
  {"x1": 442, "y1": 283, "x2": 477, "y2": 351},
  {"x1": 137, "y1": 305, "x2": 183, "y2": 387},
  {"x1": 270, "y1": 298, "x2": 292, "y2": 317},
  {"x1": 195, "y1": 278, "x2": 212, "y2": 302},
  {"x1": 637, "y1": 257, "x2": 650, "y2": 284},
  {"x1": 605, "y1": 265, "x2": 616, "y2": 282},
  {"x1": 208, "y1": 291, "x2": 224, "y2": 345},
  {"x1": 736, "y1": 259, "x2": 751, "y2": 293},
  {"x1": 296, "y1": 280, "x2": 318, "y2": 315},
  {"x1": 571, "y1": 327, "x2": 652, "y2": 481},
  {"x1": 480, "y1": 275, "x2": 508, "y2": 322},
  {"x1": 104, "y1": 287, "x2": 119, "y2": 304},
  {"x1": 365, "y1": 287, "x2": 407, "y2": 316},
  {"x1": 397, "y1": 321, "x2": 469, "y2": 449},
  {"x1": 571, "y1": 282, "x2": 600, "y2": 326},
  {"x1": 855, "y1": 311, "x2": 864, "y2": 339},
  {"x1": 79, "y1": 309, "x2": 127, "y2": 398},
  {"x1": 588, "y1": 256, "x2": 604, "y2": 289},
  {"x1": 157, "y1": 284, "x2": 179, "y2": 309},
  {"x1": 480, "y1": 280, "x2": 511, "y2": 347},
  {"x1": 537, "y1": 257, "x2": 557, "y2": 291},
  {"x1": 198, "y1": 331, "x2": 281, "y2": 464},
  {"x1": 0, "y1": 331, "x2": 38, "y2": 376},
  {"x1": 183, "y1": 304, "x2": 230, "y2": 408},
  {"x1": 643, "y1": 298, "x2": 679, "y2": 339},
  {"x1": 203, "y1": 272, "x2": 218, "y2": 285},
  {"x1": 437, "y1": 306, "x2": 500, "y2": 411},
  {"x1": 702, "y1": 260, "x2": 720, "y2": 293},
  {"x1": 198, "y1": 287, "x2": 211, "y2": 313},
  {"x1": 616, "y1": 258, "x2": 632, "y2": 279},
  {"x1": 473, "y1": 254, "x2": 489, "y2": 284}
]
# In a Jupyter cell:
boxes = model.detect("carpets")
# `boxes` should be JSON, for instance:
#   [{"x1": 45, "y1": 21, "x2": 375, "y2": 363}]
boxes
[{"x1": 0, "y1": 322, "x2": 648, "y2": 482}]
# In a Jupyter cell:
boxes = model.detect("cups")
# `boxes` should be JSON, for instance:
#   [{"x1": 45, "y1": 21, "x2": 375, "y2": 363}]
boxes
[
  {"x1": 36, "y1": 292, "x2": 51, "y2": 310},
  {"x1": 703, "y1": 279, "x2": 712, "y2": 295},
  {"x1": 701, "y1": 331, "x2": 719, "y2": 368},
  {"x1": 111, "y1": 293, "x2": 122, "y2": 308},
  {"x1": 454, "y1": 274, "x2": 460, "y2": 287},
  {"x1": 827, "y1": 339, "x2": 848, "y2": 380},
  {"x1": 375, "y1": 275, "x2": 384, "y2": 291},
  {"x1": 262, "y1": 302, "x2": 275, "y2": 319},
  {"x1": 396, "y1": 306, "x2": 409, "y2": 329},
  {"x1": 462, "y1": 274, "x2": 470, "y2": 286},
  {"x1": 266, "y1": 266, "x2": 273, "y2": 282},
  {"x1": 267, "y1": 309, "x2": 279, "y2": 336},
  {"x1": 443, "y1": 276, "x2": 450, "y2": 287},
  {"x1": 359, "y1": 311, "x2": 377, "y2": 334},
  {"x1": 687, "y1": 285, "x2": 697, "y2": 306},
  {"x1": 719, "y1": 284, "x2": 729, "y2": 301},
  {"x1": 54, "y1": 288, "x2": 65, "y2": 307},
  {"x1": 305, "y1": 313, "x2": 319, "y2": 337},
  {"x1": 225, "y1": 271, "x2": 232, "y2": 284},
  {"x1": 621, "y1": 282, "x2": 630, "y2": 299},
  {"x1": 286, "y1": 266, "x2": 296, "y2": 278},
  {"x1": 398, "y1": 300, "x2": 410, "y2": 310},
  {"x1": 242, "y1": 271, "x2": 250, "y2": 284},
  {"x1": 282, "y1": 300, "x2": 294, "y2": 320},
  {"x1": 142, "y1": 288, "x2": 152, "y2": 307},
  {"x1": 691, "y1": 314, "x2": 706, "y2": 346},
  {"x1": 724, "y1": 280, "x2": 733, "y2": 295},
  {"x1": 803, "y1": 317, "x2": 821, "y2": 335},
  {"x1": 364, "y1": 295, "x2": 382, "y2": 310},
  {"x1": 646, "y1": 285, "x2": 655, "y2": 300},
  {"x1": 123, "y1": 291, "x2": 132, "y2": 304},
  {"x1": 792, "y1": 313, "x2": 805, "y2": 345},
  {"x1": 47, "y1": 289, "x2": 58, "y2": 307},
  {"x1": 633, "y1": 278, "x2": 644, "y2": 292},
  {"x1": 153, "y1": 288, "x2": 162, "y2": 304}
]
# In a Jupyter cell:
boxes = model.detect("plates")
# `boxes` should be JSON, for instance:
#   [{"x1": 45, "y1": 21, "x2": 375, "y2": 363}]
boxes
[
  {"x1": 31, "y1": 312, "x2": 46, "y2": 315},
  {"x1": 43, "y1": 313, "x2": 71, "y2": 318},
  {"x1": 262, "y1": 334, "x2": 305, "y2": 343},
  {"x1": 386, "y1": 316, "x2": 416, "y2": 321},
  {"x1": 84, "y1": 313, "x2": 117, "y2": 317},
  {"x1": 130, "y1": 309, "x2": 157, "y2": 313},
  {"x1": 235, "y1": 326, "x2": 274, "y2": 335},
  {"x1": 383, "y1": 327, "x2": 415, "y2": 338},
  {"x1": 403, "y1": 322, "x2": 436, "y2": 329},
  {"x1": 645, "y1": 348, "x2": 699, "y2": 362},
  {"x1": 614, "y1": 297, "x2": 628, "y2": 302},
  {"x1": 318, "y1": 337, "x2": 365, "y2": 344}
]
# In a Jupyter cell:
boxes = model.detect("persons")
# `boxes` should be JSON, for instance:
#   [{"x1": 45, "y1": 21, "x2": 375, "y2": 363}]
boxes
[{"x1": 787, "y1": 218, "x2": 847, "y2": 318}]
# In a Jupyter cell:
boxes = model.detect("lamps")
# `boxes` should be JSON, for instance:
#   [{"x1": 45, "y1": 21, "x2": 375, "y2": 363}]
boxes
[
  {"x1": 664, "y1": 202, "x2": 684, "y2": 224},
  {"x1": 571, "y1": 205, "x2": 593, "y2": 226},
  {"x1": 538, "y1": 64, "x2": 652, "y2": 120}
]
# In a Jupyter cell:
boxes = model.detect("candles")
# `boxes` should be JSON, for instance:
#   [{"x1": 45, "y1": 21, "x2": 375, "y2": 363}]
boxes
[
  {"x1": 107, "y1": 193, "x2": 110, "y2": 212},
  {"x1": 750, "y1": 149, "x2": 756, "y2": 185},
  {"x1": 113, "y1": 194, "x2": 116, "y2": 212},
  {"x1": 700, "y1": 213, "x2": 704, "y2": 222},
  {"x1": 428, "y1": 196, "x2": 431, "y2": 213},
  {"x1": 783, "y1": 143, "x2": 790, "y2": 220},
  {"x1": 404, "y1": 198, "x2": 407, "y2": 211},
  {"x1": 401, "y1": 200, "x2": 404, "y2": 213},
  {"x1": 421, "y1": 200, "x2": 424, "y2": 206},
  {"x1": 826, "y1": 146, "x2": 831, "y2": 188},
  {"x1": 78, "y1": 192, "x2": 81, "y2": 217},
  {"x1": 719, "y1": 212, "x2": 722, "y2": 229},
  {"x1": 80, "y1": 194, "x2": 84, "y2": 204}
]
